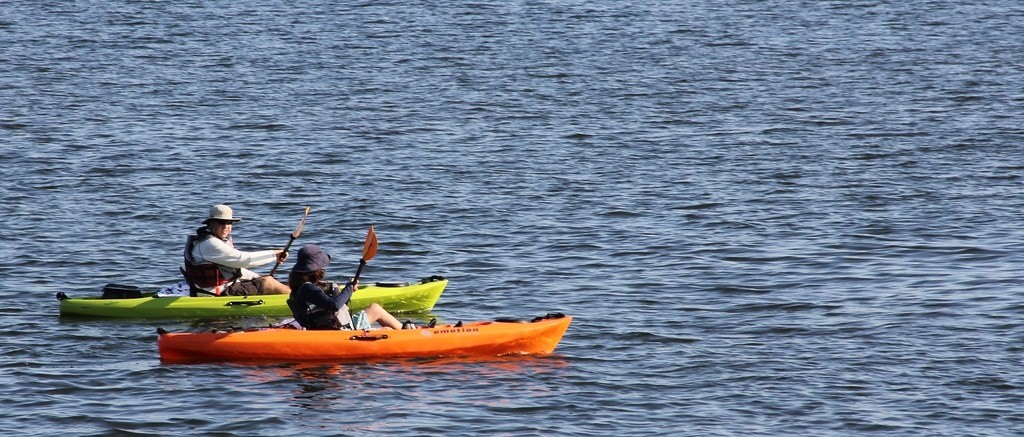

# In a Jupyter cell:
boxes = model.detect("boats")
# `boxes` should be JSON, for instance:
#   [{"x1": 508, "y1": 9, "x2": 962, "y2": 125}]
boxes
[
  {"x1": 157, "y1": 313, "x2": 572, "y2": 363},
  {"x1": 55, "y1": 272, "x2": 449, "y2": 320}
]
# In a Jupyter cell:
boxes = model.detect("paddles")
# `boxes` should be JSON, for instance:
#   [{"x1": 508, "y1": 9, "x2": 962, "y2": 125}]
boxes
[
  {"x1": 270, "y1": 207, "x2": 311, "y2": 276},
  {"x1": 354, "y1": 226, "x2": 377, "y2": 283}
]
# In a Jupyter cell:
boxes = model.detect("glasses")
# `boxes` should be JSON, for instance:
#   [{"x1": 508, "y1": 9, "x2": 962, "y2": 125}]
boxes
[{"x1": 212, "y1": 220, "x2": 234, "y2": 224}]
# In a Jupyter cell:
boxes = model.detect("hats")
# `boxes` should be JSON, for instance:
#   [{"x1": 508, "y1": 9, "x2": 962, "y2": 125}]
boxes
[
  {"x1": 202, "y1": 204, "x2": 241, "y2": 225},
  {"x1": 292, "y1": 245, "x2": 332, "y2": 273}
]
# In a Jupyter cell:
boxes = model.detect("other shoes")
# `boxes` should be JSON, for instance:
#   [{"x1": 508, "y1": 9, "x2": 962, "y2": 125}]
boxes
[
  {"x1": 428, "y1": 318, "x2": 437, "y2": 328},
  {"x1": 454, "y1": 321, "x2": 462, "y2": 327}
]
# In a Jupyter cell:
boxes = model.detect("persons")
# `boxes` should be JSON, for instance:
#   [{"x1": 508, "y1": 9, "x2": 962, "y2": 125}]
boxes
[
  {"x1": 286, "y1": 244, "x2": 462, "y2": 332},
  {"x1": 184, "y1": 204, "x2": 291, "y2": 297}
]
